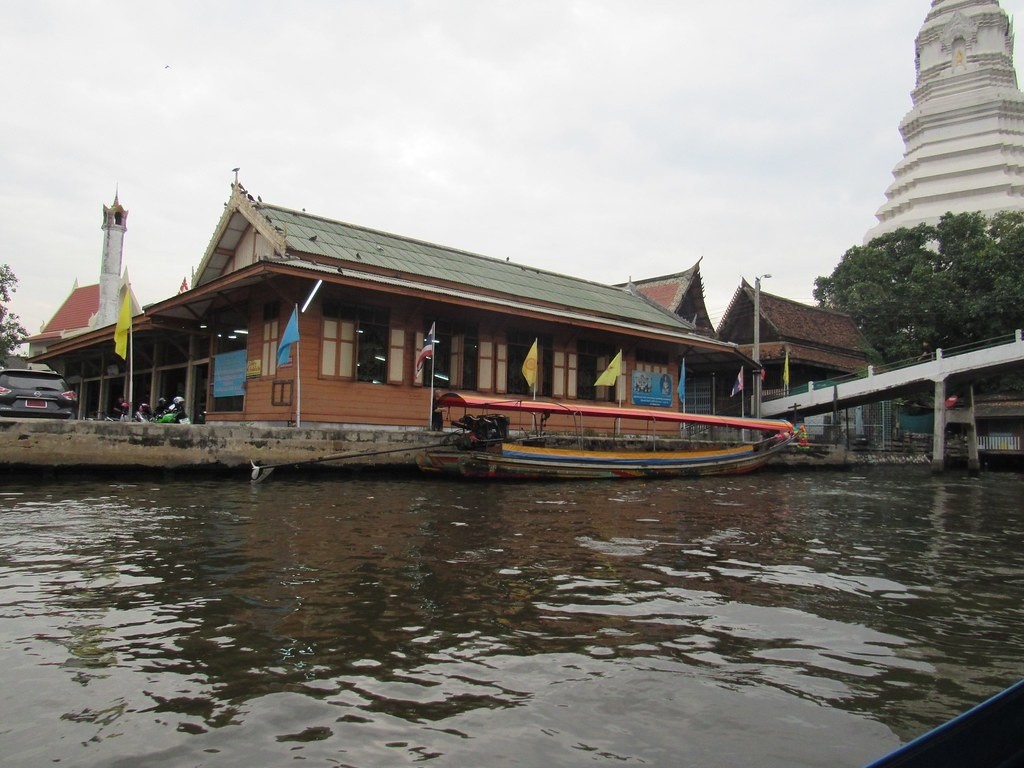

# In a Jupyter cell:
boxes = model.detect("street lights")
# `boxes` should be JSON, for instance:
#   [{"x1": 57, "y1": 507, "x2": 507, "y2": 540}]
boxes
[{"x1": 754, "y1": 273, "x2": 772, "y2": 418}]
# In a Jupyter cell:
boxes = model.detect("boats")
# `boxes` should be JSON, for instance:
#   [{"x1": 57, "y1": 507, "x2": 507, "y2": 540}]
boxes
[{"x1": 412, "y1": 392, "x2": 797, "y2": 478}]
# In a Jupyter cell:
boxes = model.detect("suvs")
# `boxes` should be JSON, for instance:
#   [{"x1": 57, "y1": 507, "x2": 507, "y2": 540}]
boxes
[{"x1": 0, "y1": 367, "x2": 77, "y2": 421}]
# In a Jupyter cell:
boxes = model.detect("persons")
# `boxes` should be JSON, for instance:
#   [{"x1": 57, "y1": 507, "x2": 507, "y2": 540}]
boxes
[
  {"x1": 636, "y1": 376, "x2": 649, "y2": 392},
  {"x1": 114, "y1": 399, "x2": 129, "y2": 421}
]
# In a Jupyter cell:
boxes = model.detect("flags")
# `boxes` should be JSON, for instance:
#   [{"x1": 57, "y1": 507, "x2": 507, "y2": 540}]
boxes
[
  {"x1": 677, "y1": 359, "x2": 685, "y2": 402},
  {"x1": 730, "y1": 367, "x2": 743, "y2": 397},
  {"x1": 114, "y1": 289, "x2": 130, "y2": 360},
  {"x1": 416, "y1": 326, "x2": 433, "y2": 377},
  {"x1": 521, "y1": 340, "x2": 537, "y2": 387},
  {"x1": 783, "y1": 352, "x2": 789, "y2": 386},
  {"x1": 277, "y1": 308, "x2": 300, "y2": 367},
  {"x1": 594, "y1": 352, "x2": 621, "y2": 386}
]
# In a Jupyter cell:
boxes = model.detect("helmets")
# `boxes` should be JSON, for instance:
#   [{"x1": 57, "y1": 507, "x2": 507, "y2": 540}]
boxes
[
  {"x1": 120, "y1": 402, "x2": 129, "y2": 410},
  {"x1": 159, "y1": 398, "x2": 166, "y2": 403},
  {"x1": 173, "y1": 396, "x2": 185, "y2": 404},
  {"x1": 138, "y1": 403, "x2": 150, "y2": 412}
]
